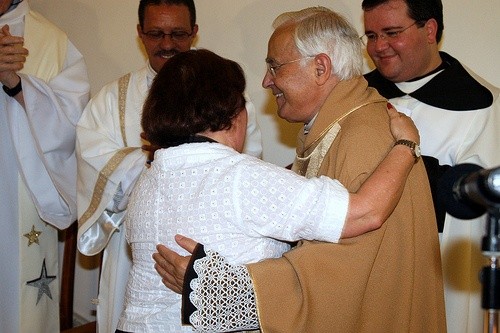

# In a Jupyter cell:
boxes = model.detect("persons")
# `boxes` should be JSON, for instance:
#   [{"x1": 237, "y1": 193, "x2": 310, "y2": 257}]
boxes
[
  {"x1": 360, "y1": 0, "x2": 500, "y2": 333},
  {"x1": 76, "y1": 0, "x2": 263, "y2": 333},
  {"x1": 152, "y1": 7, "x2": 449, "y2": 333},
  {"x1": 0, "y1": 0, "x2": 91, "y2": 333},
  {"x1": 113, "y1": 49, "x2": 420, "y2": 333}
]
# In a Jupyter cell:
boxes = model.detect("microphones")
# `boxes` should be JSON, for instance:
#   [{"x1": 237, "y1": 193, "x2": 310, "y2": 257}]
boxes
[{"x1": 439, "y1": 163, "x2": 500, "y2": 220}]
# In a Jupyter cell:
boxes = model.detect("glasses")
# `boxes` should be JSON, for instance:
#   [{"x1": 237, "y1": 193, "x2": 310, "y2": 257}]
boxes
[
  {"x1": 265, "y1": 56, "x2": 316, "y2": 78},
  {"x1": 140, "y1": 29, "x2": 195, "y2": 42},
  {"x1": 359, "y1": 22, "x2": 417, "y2": 47}
]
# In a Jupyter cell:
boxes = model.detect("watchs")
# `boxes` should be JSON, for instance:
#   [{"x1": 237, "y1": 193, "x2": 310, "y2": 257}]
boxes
[
  {"x1": 1, "y1": 76, "x2": 22, "y2": 97},
  {"x1": 392, "y1": 140, "x2": 421, "y2": 164}
]
